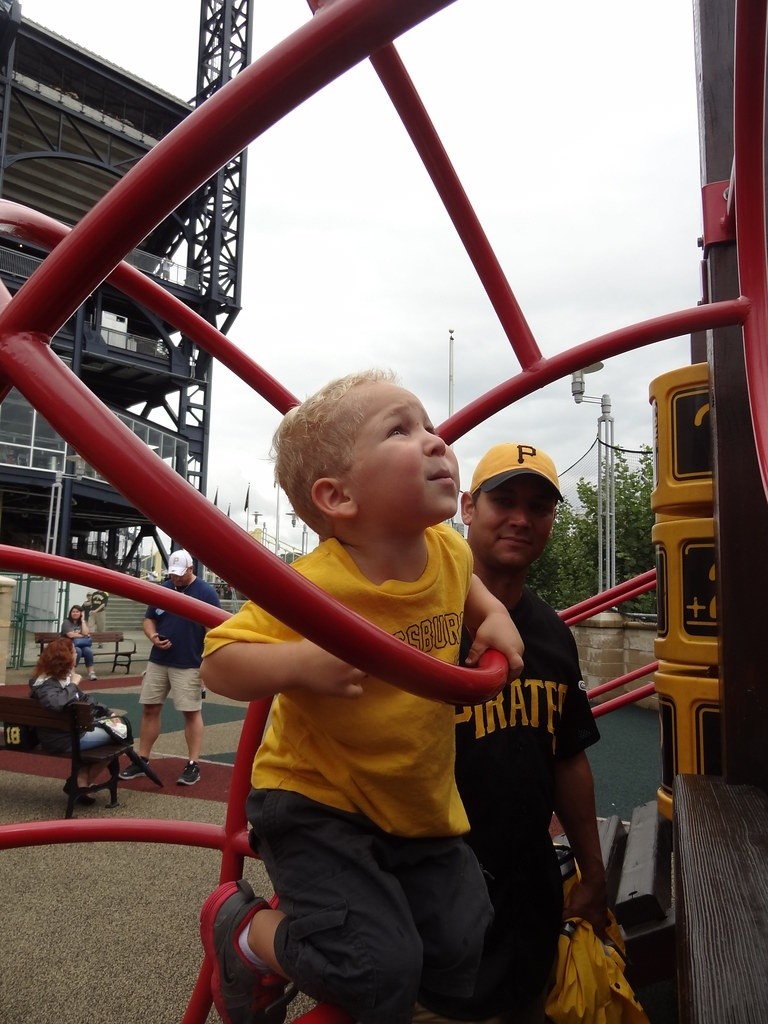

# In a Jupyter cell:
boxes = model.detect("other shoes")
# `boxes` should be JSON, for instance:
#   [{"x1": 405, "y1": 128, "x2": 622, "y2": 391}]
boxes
[{"x1": 64, "y1": 776, "x2": 95, "y2": 807}]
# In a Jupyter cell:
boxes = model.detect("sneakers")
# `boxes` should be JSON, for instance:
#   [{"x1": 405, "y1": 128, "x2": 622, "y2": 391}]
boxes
[
  {"x1": 88, "y1": 670, "x2": 97, "y2": 680},
  {"x1": 177, "y1": 761, "x2": 200, "y2": 785},
  {"x1": 200, "y1": 879, "x2": 291, "y2": 1024},
  {"x1": 119, "y1": 761, "x2": 152, "y2": 779}
]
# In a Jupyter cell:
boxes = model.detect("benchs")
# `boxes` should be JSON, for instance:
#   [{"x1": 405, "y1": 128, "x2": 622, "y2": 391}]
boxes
[
  {"x1": 0, "y1": 696, "x2": 134, "y2": 820},
  {"x1": 34, "y1": 631, "x2": 137, "y2": 675}
]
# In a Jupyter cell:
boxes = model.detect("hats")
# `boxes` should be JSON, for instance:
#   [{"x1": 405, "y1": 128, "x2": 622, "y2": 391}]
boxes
[
  {"x1": 168, "y1": 549, "x2": 193, "y2": 576},
  {"x1": 469, "y1": 442, "x2": 564, "y2": 503}
]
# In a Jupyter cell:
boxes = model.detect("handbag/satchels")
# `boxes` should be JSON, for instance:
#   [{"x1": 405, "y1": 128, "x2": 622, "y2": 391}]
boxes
[{"x1": 73, "y1": 636, "x2": 92, "y2": 646}]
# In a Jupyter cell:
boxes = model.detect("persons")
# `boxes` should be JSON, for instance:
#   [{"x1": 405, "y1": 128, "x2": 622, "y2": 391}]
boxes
[
  {"x1": 118, "y1": 549, "x2": 222, "y2": 785},
  {"x1": 199, "y1": 369, "x2": 513, "y2": 1024},
  {"x1": 60, "y1": 606, "x2": 98, "y2": 681},
  {"x1": 453, "y1": 445, "x2": 608, "y2": 1024},
  {"x1": 148, "y1": 566, "x2": 158, "y2": 582},
  {"x1": 81, "y1": 590, "x2": 108, "y2": 648},
  {"x1": 28, "y1": 637, "x2": 128, "y2": 807}
]
